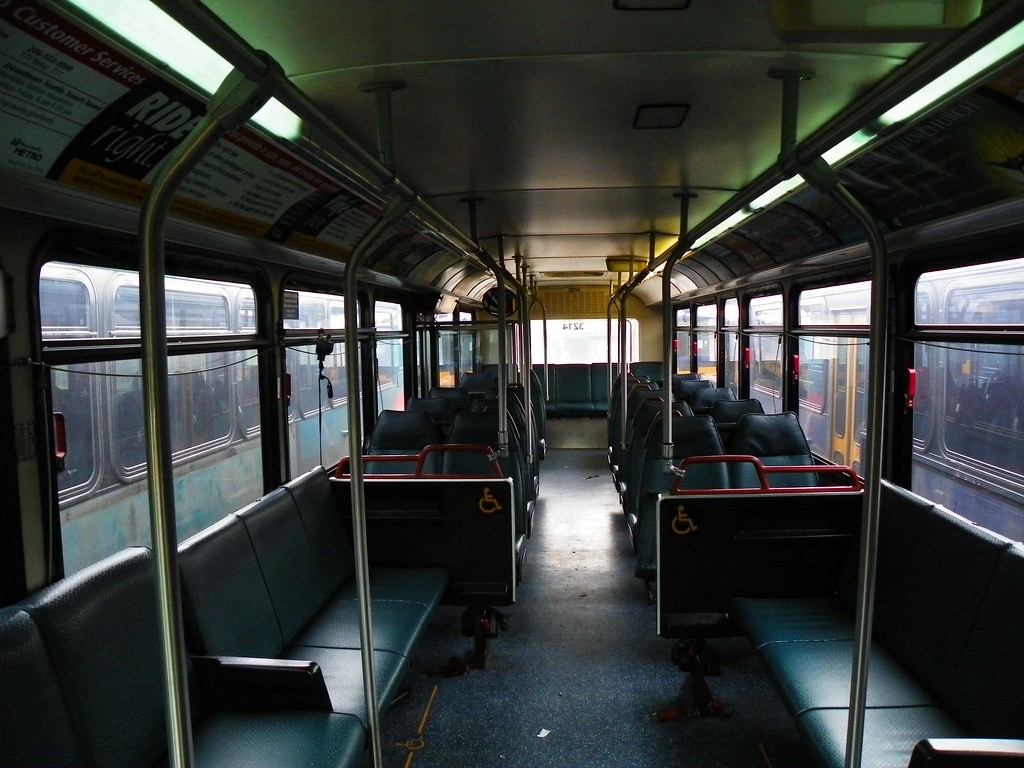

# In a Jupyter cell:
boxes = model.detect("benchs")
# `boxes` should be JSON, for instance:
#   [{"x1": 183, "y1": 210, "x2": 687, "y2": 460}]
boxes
[{"x1": 0, "y1": 362, "x2": 1024, "y2": 768}]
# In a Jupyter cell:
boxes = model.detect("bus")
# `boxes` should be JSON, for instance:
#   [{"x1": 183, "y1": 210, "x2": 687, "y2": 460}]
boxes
[
  {"x1": 678, "y1": 257, "x2": 1024, "y2": 546},
  {"x1": 39, "y1": 261, "x2": 476, "y2": 581}
]
